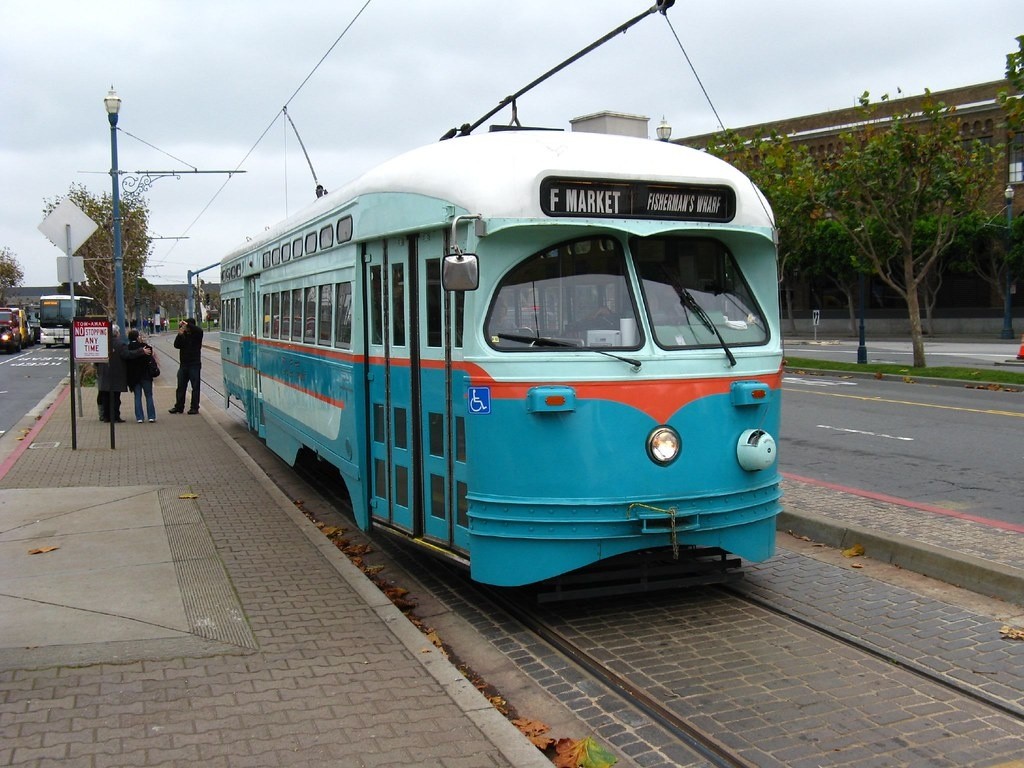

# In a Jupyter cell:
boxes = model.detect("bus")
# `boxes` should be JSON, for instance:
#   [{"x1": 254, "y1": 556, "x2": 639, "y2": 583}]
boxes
[
  {"x1": 35, "y1": 295, "x2": 109, "y2": 349},
  {"x1": 223, "y1": 132, "x2": 785, "y2": 605}
]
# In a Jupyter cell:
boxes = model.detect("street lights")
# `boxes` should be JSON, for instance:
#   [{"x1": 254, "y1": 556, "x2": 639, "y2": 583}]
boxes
[
  {"x1": 103, "y1": 83, "x2": 123, "y2": 344},
  {"x1": 1002, "y1": 185, "x2": 1015, "y2": 340}
]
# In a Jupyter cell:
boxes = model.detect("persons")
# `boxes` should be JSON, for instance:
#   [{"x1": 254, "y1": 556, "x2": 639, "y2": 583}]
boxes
[
  {"x1": 149, "y1": 317, "x2": 169, "y2": 334},
  {"x1": 127, "y1": 330, "x2": 157, "y2": 423},
  {"x1": 168, "y1": 318, "x2": 203, "y2": 414},
  {"x1": 91, "y1": 323, "x2": 151, "y2": 423}
]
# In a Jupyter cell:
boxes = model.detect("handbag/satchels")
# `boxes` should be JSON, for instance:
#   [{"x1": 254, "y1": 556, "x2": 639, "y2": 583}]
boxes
[{"x1": 139, "y1": 342, "x2": 160, "y2": 377}]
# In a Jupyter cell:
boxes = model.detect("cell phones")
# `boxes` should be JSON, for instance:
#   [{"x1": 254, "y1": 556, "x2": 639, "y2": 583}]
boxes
[{"x1": 179, "y1": 325, "x2": 182, "y2": 329}]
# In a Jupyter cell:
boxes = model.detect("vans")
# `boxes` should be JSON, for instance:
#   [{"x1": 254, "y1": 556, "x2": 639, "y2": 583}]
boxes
[
  {"x1": 0, "y1": 308, "x2": 22, "y2": 354},
  {"x1": 19, "y1": 310, "x2": 32, "y2": 349}
]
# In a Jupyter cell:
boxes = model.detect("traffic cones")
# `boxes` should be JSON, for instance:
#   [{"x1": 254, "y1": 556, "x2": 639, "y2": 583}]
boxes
[{"x1": 1016, "y1": 332, "x2": 1024, "y2": 360}]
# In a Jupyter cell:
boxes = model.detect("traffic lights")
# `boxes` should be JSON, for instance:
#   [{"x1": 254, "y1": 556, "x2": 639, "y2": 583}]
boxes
[
  {"x1": 135, "y1": 298, "x2": 140, "y2": 306},
  {"x1": 207, "y1": 315, "x2": 210, "y2": 318},
  {"x1": 205, "y1": 293, "x2": 210, "y2": 304}
]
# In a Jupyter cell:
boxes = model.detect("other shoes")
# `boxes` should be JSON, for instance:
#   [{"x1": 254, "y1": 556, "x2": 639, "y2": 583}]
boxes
[
  {"x1": 149, "y1": 418, "x2": 154, "y2": 422},
  {"x1": 115, "y1": 419, "x2": 125, "y2": 422},
  {"x1": 104, "y1": 419, "x2": 111, "y2": 423},
  {"x1": 169, "y1": 407, "x2": 183, "y2": 413},
  {"x1": 188, "y1": 408, "x2": 199, "y2": 414},
  {"x1": 99, "y1": 416, "x2": 104, "y2": 421},
  {"x1": 138, "y1": 419, "x2": 143, "y2": 423}
]
